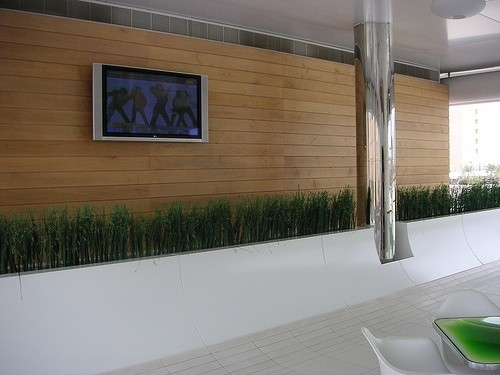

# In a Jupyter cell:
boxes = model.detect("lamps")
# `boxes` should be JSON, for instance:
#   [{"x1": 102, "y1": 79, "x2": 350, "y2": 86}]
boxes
[{"x1": 430, "y1": 0, "x2": 487, "y2": 20}]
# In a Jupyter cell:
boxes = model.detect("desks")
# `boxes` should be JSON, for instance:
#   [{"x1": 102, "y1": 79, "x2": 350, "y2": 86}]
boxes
[{"x1": 432, "y1": 314, "x2": 500, "y2": 375}]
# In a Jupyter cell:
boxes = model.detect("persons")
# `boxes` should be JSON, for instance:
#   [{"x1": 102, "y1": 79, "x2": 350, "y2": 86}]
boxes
[{"x1": 107, "y1": 84, "x2": 198, "y2": 133}]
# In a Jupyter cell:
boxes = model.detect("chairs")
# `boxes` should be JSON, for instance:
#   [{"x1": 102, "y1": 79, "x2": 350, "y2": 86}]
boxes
[
  {"x1": 359, "y1": 325, "x2": 454, "y2": 375},
  {"x1": 435, "y1": 288, "x2": 500, "y2": 375}
]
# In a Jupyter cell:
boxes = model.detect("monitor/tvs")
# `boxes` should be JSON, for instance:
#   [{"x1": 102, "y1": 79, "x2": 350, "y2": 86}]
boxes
[{"x1": 92, "y1": 61, "x2": 210, "y2": 144}]
